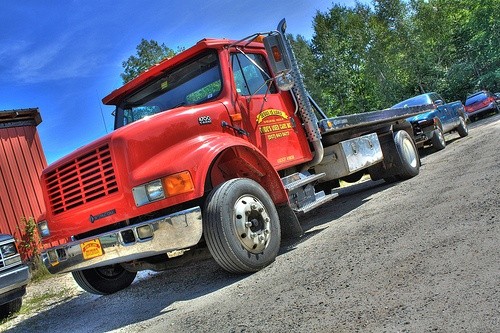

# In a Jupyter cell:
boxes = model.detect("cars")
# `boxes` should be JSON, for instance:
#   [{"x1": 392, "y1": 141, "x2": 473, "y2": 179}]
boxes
[
  {"x1": 0, "y1": 234, "x2": 30, "y2": 314},
  {"x1": 465, "y1": 90, "x2": 499, "y2": 123}
]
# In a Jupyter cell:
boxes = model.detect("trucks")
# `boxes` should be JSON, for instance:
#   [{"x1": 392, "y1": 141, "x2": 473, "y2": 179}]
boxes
[{"x1": 37, "y1": 17, "x2": 435, "y2": 296}]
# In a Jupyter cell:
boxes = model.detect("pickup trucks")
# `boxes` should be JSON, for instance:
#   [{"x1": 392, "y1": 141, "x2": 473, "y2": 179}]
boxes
[{"x1": 390, "y1": 92, "x2": 468, "y2": 152}]
